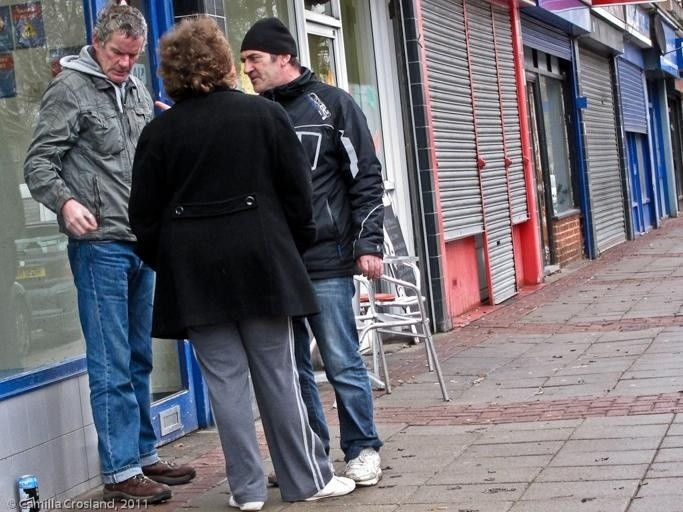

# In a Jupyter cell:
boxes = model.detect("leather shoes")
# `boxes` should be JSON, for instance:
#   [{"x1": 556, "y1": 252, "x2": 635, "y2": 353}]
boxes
[
  {"x1": 303, "y1": 475, "x2": 356, "y2": 502},
  {"x1": 104, "y1": 474, "x2": 173, "y2": 504},
  {"x1": 141, "y1": 459, "x2": 196, "y2": 485}
]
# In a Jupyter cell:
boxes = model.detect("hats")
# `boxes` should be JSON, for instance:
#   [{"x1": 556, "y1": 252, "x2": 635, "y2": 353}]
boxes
[{"x1": 240, "y1": 17, "x2": 297, "y2": 56}]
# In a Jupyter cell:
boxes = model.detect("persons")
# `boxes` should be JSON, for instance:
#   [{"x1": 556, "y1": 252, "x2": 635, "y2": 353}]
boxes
[
  {"x1": 125, "y1": 12, "x2": 357, "y2": 511},
  {"x1": 22, "y1": 4, "x2": 197, "y2": 504},
  {"x1": 237, "y1": 16, "x2": 386, "y2": 494}
]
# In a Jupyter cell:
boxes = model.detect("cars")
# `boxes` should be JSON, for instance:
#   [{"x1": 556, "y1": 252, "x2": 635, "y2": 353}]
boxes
[{"x1": 11, "y1": 282, "x2": 35, "y2": 360}]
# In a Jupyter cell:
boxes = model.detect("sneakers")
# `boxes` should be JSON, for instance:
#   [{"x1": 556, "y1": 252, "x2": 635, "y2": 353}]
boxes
[
  {"x1": 345, "y1": 447, "x2": 382, "y2": 487},
  {"x1": 228, "y1": 494, "x2": 264, "y2": 511},
  {"x1": 268, "y1": 462, "x2": 335, "y2": 485}
]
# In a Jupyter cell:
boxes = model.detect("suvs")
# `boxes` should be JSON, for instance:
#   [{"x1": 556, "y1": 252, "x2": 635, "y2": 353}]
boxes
[{"x1": 14, "y1": 218, "x2": 84, "y2": 340}]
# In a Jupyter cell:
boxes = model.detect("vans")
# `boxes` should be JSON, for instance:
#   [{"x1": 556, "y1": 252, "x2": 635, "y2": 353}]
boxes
[{"x1": 17, "y1": 182, "x2": 58, "y2": 227}]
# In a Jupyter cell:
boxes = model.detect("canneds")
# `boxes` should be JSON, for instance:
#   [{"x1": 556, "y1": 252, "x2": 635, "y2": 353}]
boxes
[{"x1": 16, "y1": 475, "x2": 40, "y2": 512}]
